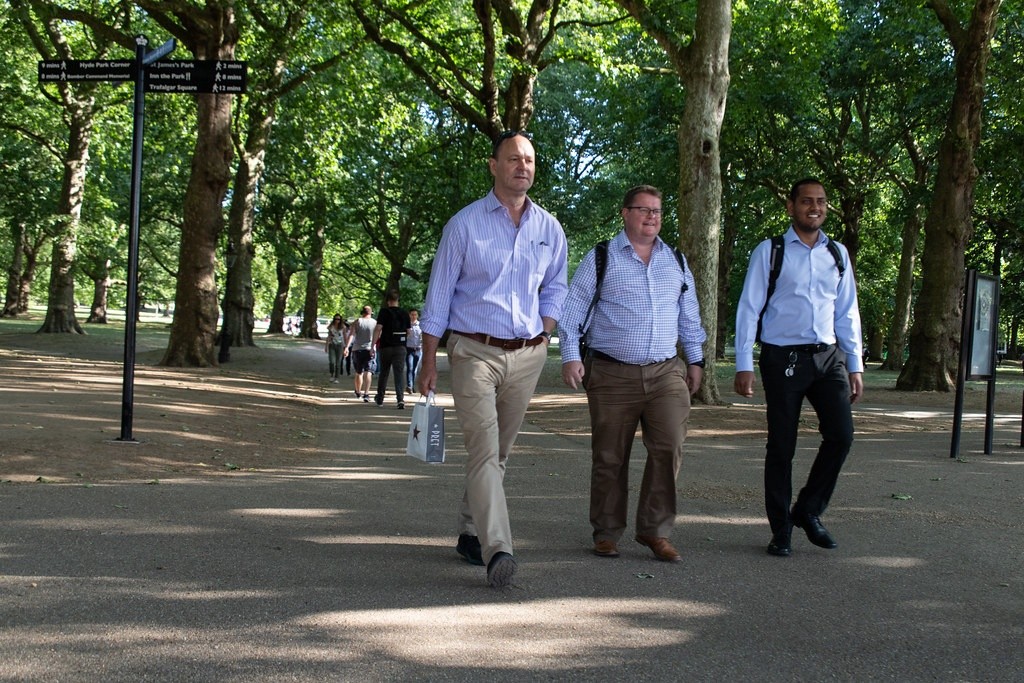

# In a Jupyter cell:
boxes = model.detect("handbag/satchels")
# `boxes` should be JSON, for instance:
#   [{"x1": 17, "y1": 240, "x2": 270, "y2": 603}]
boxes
[{"x1": 406, "y1": 391, "x2": 446, "y2": 464}]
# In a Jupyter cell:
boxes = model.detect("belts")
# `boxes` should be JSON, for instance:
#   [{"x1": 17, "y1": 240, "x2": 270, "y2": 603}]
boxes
[
  {"x1": 452, "y1": 329, "x2": 544, "y2": 350},
  {"x1": 590, "y1": 350, "x2": 678, "y2": 366},
  {"x1": 763, "y1": 343, "x2": 828, "y2": 354}
]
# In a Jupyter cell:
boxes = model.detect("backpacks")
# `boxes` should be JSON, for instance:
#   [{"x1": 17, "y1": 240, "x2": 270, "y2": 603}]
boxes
[{"x1": 380, "y1": 307, "x2": 408, "y2": 346}]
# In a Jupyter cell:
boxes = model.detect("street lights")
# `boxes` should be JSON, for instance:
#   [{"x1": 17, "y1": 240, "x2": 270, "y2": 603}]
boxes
[{"x1": 217, "y1": 238, "x2": 240, "y2": 364}]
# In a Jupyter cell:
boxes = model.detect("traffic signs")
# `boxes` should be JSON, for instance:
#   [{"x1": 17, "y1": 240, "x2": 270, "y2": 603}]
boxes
[
  {"x1": 143, "y1": 38, "x2": 176, "y2": 67},
  {"x1": 37, "y1": 59, "x2": 136, "y2": 82},
  {"x1": 144, "y1": 59, "x2": 248, "y2": 94}
]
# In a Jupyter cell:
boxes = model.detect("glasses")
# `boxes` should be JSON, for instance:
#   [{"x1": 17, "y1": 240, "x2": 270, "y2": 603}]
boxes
[
  {"x1": 626, "y1": 206, "x2": 664, "y2": 217},
  {"x1": 334, "y1": 318, "x2": 341, "y2": 321}
]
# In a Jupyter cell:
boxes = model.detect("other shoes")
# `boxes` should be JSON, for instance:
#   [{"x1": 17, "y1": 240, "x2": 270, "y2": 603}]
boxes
[
  {"x1": 397, "y1": 401, "x2": 406, "y2": 409},
  {"x1": 334, "y1": 380, "x2": 340, "y2": 384},
  {"x1": 406, "y1": 386, "x2": 414, "y2": 395},
  {"x1": 375, "y1": 395, "x2": 383, "y2": 405},
  {"x1": 354, "y1": 390, "x2": 361, "y2": 397},
  {"x1": 363, "y1": 395, "x2": 369, "y2": 401},
  {"x1": 330, "y1": 377, "x2": 334, "y2": 381}
]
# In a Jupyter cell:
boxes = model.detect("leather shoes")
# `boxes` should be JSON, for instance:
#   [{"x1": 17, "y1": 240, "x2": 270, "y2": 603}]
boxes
[
  {"x1": 634, "y1": 534, "x2": 681, "y2": 562},
  {"x1": 791, "y1": 510, "x2": 837, "y2": 549},
  {"x1": 485, "y1": 552, "x2": 518, "y2": 587},
  {"x1": 595, "y1": 539, "x2": 619, "y2": 557},
  {"x1": 456, "y1": 534, "x2": 486, "y2": 565},
  {"x1": 767, "y1": 533, "x2": 792, "y2": 555}
]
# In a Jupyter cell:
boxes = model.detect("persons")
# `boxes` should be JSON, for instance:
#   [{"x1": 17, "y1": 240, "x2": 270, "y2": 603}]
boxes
[
  {"x1": 264, "y1": 317, "x2": 269, "y2": 322},
  {"x1": 734, "y1": 177, "x2": 865, "y2": 557},
  {"x1": 325, "y1": 292, "x2": 422, "y2": 409},
  {"x1": 416, "y1": 134, "x2": 568, "y2": 586},
  {"x1": 557, "y1": 185, "x2": 707, "y2": 560},
  {"x1": 286, "y1": 317, "x2": 304, "y2": 334}
]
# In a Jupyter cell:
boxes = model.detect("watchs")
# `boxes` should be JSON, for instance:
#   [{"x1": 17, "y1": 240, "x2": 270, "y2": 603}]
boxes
[
  {"x1": 539, "y1": 331, "x2": 552, "y2": 344},
  {"x1": 688, "y1": 357, "x2": 706, "y2": 368}
]
